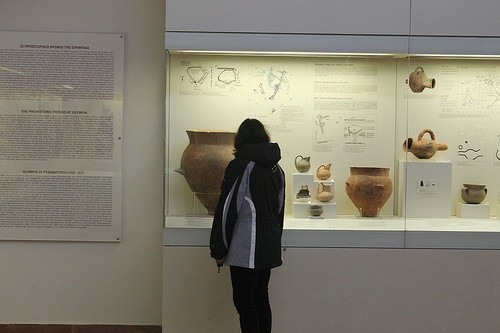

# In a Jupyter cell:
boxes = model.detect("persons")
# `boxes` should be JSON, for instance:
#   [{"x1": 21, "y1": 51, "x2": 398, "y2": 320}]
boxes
[{"x1": 209, "y1": 118, "x2": 286, "y2": 333}]
